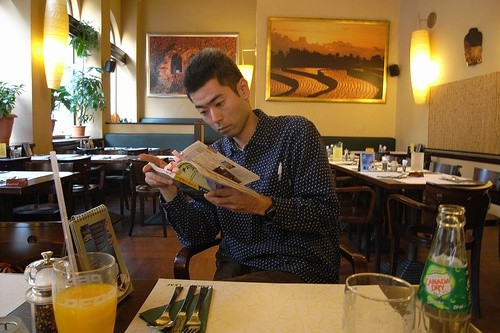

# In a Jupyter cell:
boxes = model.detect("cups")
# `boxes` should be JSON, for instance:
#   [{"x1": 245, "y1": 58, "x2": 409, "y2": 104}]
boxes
[
  {"x1": 52, "y1": 252, "x2": 118, "y2": 333},
  {"x1": 343, "y1": 273, "x2": 415, "y2": 333},
  {"x1": 411, "y1": 152, "x2": 424, "y2": 172},
  {"x1": 333, "y1": 147, "x2": 342, "y2": 161}
]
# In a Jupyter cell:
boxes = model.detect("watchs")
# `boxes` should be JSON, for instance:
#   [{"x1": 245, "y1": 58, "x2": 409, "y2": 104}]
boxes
[{"x1": 265, "y1": 196, "x2": 277, "y2": 218}]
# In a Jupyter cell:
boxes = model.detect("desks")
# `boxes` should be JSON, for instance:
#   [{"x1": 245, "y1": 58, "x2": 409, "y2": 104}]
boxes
[
  {"x1": 328, "y1": 155, "x2": 486, "y2": 274},
  {"x1": 0, "y1": 147, "x2": 171, "y2": 217},
  {"x1": 0, "y1": 273, "x2": 481, "y2": 333}
]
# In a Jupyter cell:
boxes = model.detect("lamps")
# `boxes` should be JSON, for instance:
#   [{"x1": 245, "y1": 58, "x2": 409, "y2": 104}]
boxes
[
  {"x1": 236, "y1": 45, "x2": 257, "y2": 90},
  {"x1": 43, "y1": 0, "x2": 69, "y2": 90},
  {"x1": 409, "y1": 11, "x2": 438, "y2": 105}
]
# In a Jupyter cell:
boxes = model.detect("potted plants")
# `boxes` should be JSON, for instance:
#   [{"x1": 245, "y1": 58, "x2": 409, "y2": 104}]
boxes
[
  {"x1": 53, "y1": 19, "x2": 107, "y2": 136},
  {"x1": 0, "y1": 80, "x2": 25, "y2": 144},
  {"x1": 51, "y1": 86, "x2": 72, "y2": 132}
]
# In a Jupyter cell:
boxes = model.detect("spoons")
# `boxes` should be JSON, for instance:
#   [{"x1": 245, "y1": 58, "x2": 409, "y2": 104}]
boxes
[{"x1": 147, "y1": 286, "x2": 184, "y2": 328}]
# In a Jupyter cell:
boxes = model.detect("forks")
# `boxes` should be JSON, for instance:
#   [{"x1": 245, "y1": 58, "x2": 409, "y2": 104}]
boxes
[{"x1": 182, "y1": 285, "x2": 209, "y2": 333}]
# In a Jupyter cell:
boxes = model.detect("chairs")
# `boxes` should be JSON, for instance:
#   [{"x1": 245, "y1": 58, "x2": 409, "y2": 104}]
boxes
[
  {"x1": 174, "y1": 163, "x2": 500, "y2": 320},
  {"x1": 127, "y1": 158, "x2": 168, "y2": 237},
  {"x1": 0, "y1": 221, "x2": 66, "y2": 273},
  {"x1": 48, "y1": 157, "x2": 105, "y2": 212}
]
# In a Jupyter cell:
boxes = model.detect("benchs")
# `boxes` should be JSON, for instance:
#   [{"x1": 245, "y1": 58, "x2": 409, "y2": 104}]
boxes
[{"x1": 105, "y1": 117, "x2": 221, "y2": 153}]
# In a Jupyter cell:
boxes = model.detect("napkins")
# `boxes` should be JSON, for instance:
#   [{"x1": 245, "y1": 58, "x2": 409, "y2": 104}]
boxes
[{"x1": 139, "y1": 288, "x2": 212, "y2": 333}]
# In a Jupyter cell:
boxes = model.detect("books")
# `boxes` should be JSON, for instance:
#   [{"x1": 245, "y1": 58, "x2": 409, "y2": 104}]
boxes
[
  {"x1": 148, "y1": 141, "x2": 262, "y2": 200},
  {"x1": 69, "y1": 204, "x2": 135, "y2": 304}
]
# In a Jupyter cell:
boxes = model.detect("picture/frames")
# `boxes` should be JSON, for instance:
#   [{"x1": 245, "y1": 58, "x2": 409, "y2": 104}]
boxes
[
  {"x1": 145, "y1": 32, "x2": 240, "y2": 98},
  {"x1": 265, "y1": 16, "x2": 390, "y2": 104}
]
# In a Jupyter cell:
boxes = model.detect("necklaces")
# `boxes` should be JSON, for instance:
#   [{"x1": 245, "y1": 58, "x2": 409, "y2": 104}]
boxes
[{"x1": 235, "y1": 143, "x2": 243, "y2": 150}]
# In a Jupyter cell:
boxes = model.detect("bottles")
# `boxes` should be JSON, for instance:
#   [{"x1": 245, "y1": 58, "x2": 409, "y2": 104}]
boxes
[
  {"x1": 23, "y1": 251, "x2": 70, "y2": 333},
  {"x1": 381, "y1": 156, "x2": 387, "y2": 171},
  {"x1": 413, "y1": 204, "x2": 472, "y2": 333}
]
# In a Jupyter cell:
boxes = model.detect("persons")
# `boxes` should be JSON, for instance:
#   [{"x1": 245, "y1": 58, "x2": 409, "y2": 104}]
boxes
[
  {"x1": 139, "y1": 51, "x2": 342, "y2": 284},
  {"x1": 464, "y1": 27, "x2": 482, "y2": 66}
]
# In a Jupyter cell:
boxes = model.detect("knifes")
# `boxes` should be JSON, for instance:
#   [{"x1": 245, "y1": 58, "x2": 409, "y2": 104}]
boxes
[{"x1": 168, "y1": 285, "x2": 197, "y2": 333}]
